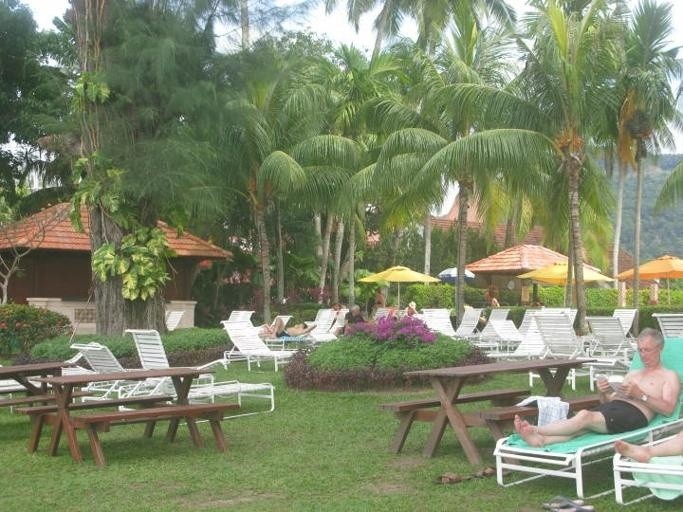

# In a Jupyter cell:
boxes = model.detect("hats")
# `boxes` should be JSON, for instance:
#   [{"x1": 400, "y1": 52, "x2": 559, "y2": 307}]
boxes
[{"x1": 408, "y1": 301, "x2": 417, "y2": 309}]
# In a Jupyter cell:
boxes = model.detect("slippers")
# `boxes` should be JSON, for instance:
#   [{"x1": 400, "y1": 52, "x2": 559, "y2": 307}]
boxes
[
  {"x1": 550, "y1": 501, "x2": 595, "y2": 512},
  {"x1": 475, "y1": 465, "x2": 513, "y2": 481},
  {"x1": 434, "y1": 469, "x2": 470, "y2": 485},
  {"x1": 541, "y1": 494, "x2": 584, "y2": 511}
]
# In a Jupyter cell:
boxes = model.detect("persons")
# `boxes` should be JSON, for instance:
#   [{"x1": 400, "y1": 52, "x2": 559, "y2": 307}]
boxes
[
  {"x1": 335, "y1": 305, "x2": 364, "y2": 336},
  {"x1": 374, "y1": 288, "x2": 385, "y2": 308},
  {"x1": 614, "y1": 432, "x2": 683, "y2": 462},
  {"x1": 263, "y1": 318, "x2": 317, "y2": 339},
  {"x1": 403, "y1": 301, "x2": 418, "y2": 317},
  {"x1": 484, "y1": 287, "x2": 500, "y2": 307},
  {"x1": 514, "y1": 328, "x2": 680, "y2": 447}
]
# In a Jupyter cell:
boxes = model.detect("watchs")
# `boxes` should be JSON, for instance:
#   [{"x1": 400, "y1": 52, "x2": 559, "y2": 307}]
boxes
[{"x1": 640, "y1": 394, "x2": 648, "y2": 402}]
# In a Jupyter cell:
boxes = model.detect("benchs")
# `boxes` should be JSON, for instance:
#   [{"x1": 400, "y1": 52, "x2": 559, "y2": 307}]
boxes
[
  {"x1": 1, "y1": 358, "x2": 242, "y2": 470},
  {"x1": 374, "y1": 355, "x2": 606, "y2": 470}
]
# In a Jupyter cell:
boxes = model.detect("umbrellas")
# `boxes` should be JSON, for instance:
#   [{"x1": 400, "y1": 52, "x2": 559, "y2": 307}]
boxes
[
  {"x1": 438, "y1": 268, "x2": 475, "y2": 279},
  {"x1": 516, "y1": 262, "x2": 616, "y2": 307},
  {"x1": 615, "y1": 255, "x2": 683, "y2": 305},
  {"x1": 357, "y1": 265, "x2": 442, "y2": 308}
]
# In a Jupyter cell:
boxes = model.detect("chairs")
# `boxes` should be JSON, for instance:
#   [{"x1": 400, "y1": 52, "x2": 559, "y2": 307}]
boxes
[
  {"x1": 393, "y1": 302, "x2": 682, "y2": 398},
  {"x1": 490, "y1": 333, "x2": 683, "y2": 504},
  {"x1": 1, "y1": 327, "x2": 276, "y2": 427},
  {"x1": 220, "y1": 302, "x2": 392, "y2": 372}
]
